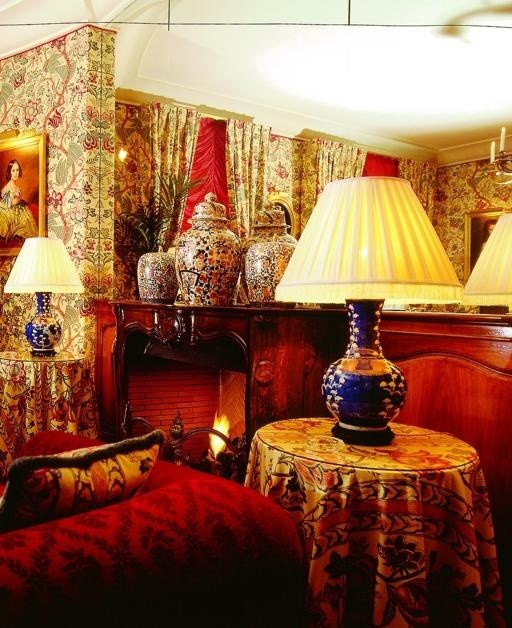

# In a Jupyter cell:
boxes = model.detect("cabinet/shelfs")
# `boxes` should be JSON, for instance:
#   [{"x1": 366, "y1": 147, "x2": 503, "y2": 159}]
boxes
[{"x1": 93, "y1": 300, "x2": 512, "y2": 592}]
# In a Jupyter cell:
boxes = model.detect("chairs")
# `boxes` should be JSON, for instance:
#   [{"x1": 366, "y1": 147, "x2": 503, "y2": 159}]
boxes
[{"x1": 0, "y1": 431, "x2": 309, "y2": 628}]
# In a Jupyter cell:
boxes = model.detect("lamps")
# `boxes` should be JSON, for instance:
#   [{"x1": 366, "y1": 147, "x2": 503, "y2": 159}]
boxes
[
  {"x1": 3, "y1": 238, "x2": 85, "y2": 356},
  {"x1": 484, "y1": 127, "x2": 512, "y2": 185},
  {"x1": 464, "y1": 215, "x2": 512, "y2": 317},
  {"x1": 273, "y1": 175, "x2": 464, "y2": 445}
]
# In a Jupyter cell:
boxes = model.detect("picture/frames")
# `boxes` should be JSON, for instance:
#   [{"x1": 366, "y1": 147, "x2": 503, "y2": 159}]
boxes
[
  {"x1": 0, "y1": 132, "x2": 47, "y2": 257},
  {"x1": 464, "y1": 212, "x2": 503, "y2": 287}
]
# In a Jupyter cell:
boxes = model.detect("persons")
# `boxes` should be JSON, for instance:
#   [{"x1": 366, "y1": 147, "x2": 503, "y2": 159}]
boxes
[
  {"x1": 474, "y1": 217, "x2": 499, "y2": 259},
  {"x1": 0, "y1": 157, "x2": 44, "y2": 243}
]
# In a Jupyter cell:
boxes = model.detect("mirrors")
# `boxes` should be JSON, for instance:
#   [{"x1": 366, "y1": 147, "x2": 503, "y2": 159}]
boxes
[
  {"x1": 116, "y1": 0, "x2": 512, "y2": 315},
  {"x1": 267, "y1": 196, "x2": 295, "y2": 238}
]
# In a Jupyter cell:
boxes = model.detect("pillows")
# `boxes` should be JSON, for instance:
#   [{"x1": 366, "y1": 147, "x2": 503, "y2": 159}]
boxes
[{"x1": 0, "y1": 428, "x2": 166, "y2": 535}]
